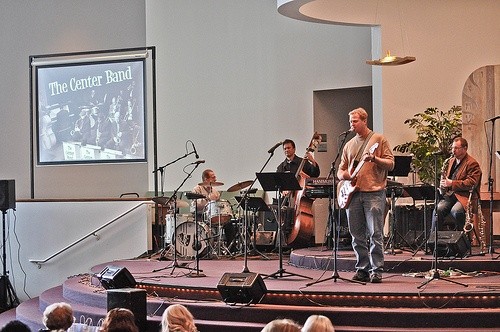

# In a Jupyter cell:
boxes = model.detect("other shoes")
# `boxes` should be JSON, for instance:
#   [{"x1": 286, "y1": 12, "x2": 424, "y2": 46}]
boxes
[
  {"x1": 370, "y1": 270, "x2": 382, "y2": 282},
  {"x1": 352, "y1": 269, "x2": 370, "y2": 281}
]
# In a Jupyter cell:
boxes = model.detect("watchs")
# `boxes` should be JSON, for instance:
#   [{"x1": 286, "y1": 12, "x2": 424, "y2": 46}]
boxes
[{"x1": 368, "y1": 156, "x2": 375, "y2": 163}]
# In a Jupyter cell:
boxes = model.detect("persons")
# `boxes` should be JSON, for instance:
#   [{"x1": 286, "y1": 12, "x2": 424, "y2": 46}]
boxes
[
  {"x1": 426, "y1": 137, "x2": 482, "y2": 255},
  {"x1": 189, "y1": 168, "x2": 237, "y2": 259},
  {"x1": 260, "y1": 318, "x2": 301, "y2": 332},
  {"x1": 276, "y1": 138, "x2": 320, "y2": 254},
  {"x1": 300, "y1": 314, "x2": 335, "y2": 332},
  {"x1": 0, "y1": 319, "x2": 32, "y2": 332},
  {"x1": 99, "y1": 307, "x2": 139, "y2": 332},
  {"x1": 51, "y1": 80, "x2": 141, "y2": 156},
  {"x1": 336, "y1": 107, "x2": 395, "y2": 284},
  {"x1": 39, "y1": 301, "x2": 73, "y2": 332},
  {"x1": 159, "y1": 304, "x2": 201, "y2": 332}
]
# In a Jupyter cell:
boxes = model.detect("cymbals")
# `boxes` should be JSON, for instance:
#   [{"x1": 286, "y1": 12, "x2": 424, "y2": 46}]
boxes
[
  {"x1": 198, "y1": 181, "x2": 225, "y2": 186},
  {"x1": 227, "y1": 180, "x2": 254, "y2": 192},
  {"x1": 152, "y1": 197, "x2": 189, "y2": 208}
]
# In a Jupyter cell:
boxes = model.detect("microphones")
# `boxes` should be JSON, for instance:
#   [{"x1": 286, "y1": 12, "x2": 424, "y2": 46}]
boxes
[
  {"x1": 192, "y1": 144, "x2": 199, "y2": 159},
  {"x1": 339, "y1": 128, "x2": 353, "y2": 136},
  {"x1": 191, "y1": 160, "x2": 205, "y2": 164},
  {"x1": 268, "y1": 141, "x2": 282, "y2": 153},
  {"x1": 485, "y1": 115, "x2": 500, "y2": 122}
]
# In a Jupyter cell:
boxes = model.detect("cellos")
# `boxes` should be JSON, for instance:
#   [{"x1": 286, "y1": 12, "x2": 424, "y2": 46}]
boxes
[{"x1": 280, "y1": 131, "x2": 323, "y2": 247}]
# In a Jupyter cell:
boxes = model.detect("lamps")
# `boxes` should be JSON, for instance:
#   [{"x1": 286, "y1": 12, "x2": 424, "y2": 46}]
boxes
[{"x1": 364, "y1": 0, "x2": 416, "y2": 66}]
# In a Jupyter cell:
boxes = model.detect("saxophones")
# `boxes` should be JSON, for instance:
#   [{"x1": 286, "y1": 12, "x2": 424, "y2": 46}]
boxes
[
  {"x1": 441, "y1": 152, "x2": 457, "y2": 196},
  {"x1": 478, "y1": 199, "x2": 487, "y2": 254},
  {"x1": 464, "y1": 184, "x2": 475, "y2": 245}
]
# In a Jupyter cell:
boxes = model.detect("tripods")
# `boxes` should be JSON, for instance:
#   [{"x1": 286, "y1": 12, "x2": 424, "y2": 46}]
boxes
[{"x1": 151, "y1": 126, "x2": 500, "y2": 289}]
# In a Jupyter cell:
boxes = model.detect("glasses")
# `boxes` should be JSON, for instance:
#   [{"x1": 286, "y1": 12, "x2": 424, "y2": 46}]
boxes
[{"x1": 451, "y1": 145, "x2": 463, "y2": 149}]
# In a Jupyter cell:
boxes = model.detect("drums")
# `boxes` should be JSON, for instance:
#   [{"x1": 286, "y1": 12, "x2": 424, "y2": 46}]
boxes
[
  {"x1": 171, "y1": 220, "x2": 214, "y2": 260},
  {"x1": 203, "y1": 198, "x2": 232, "y2": 225},
  {"x1": 165, "y1": 212, "x2": 195, "y2": 244}
]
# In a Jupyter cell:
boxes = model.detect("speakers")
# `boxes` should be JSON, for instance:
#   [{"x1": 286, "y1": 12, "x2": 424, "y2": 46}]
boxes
[
  {"x1": 260, "y1": 204, "x2": 278, "y2": 231},
  {"x1": 217, "y1": 273, "x2": 267, "y2": 304},
  {"x1": 427, "y1": 231, "x2": 470, "y2": 257},
  {"x1": 0, "y1": 179, "x2": 15, "y2": 210},
  {"x1": 105, "y1": 288, "x2": 147, "y2": 315},
  {"x1": 99, "y1": 264, "x2": 136, "y2": 290}
]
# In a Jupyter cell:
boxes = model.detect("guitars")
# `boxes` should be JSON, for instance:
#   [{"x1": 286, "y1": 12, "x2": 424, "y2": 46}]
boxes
[{"x1": 337, "y1": 142, "x2": 380, "y2": 209}]
